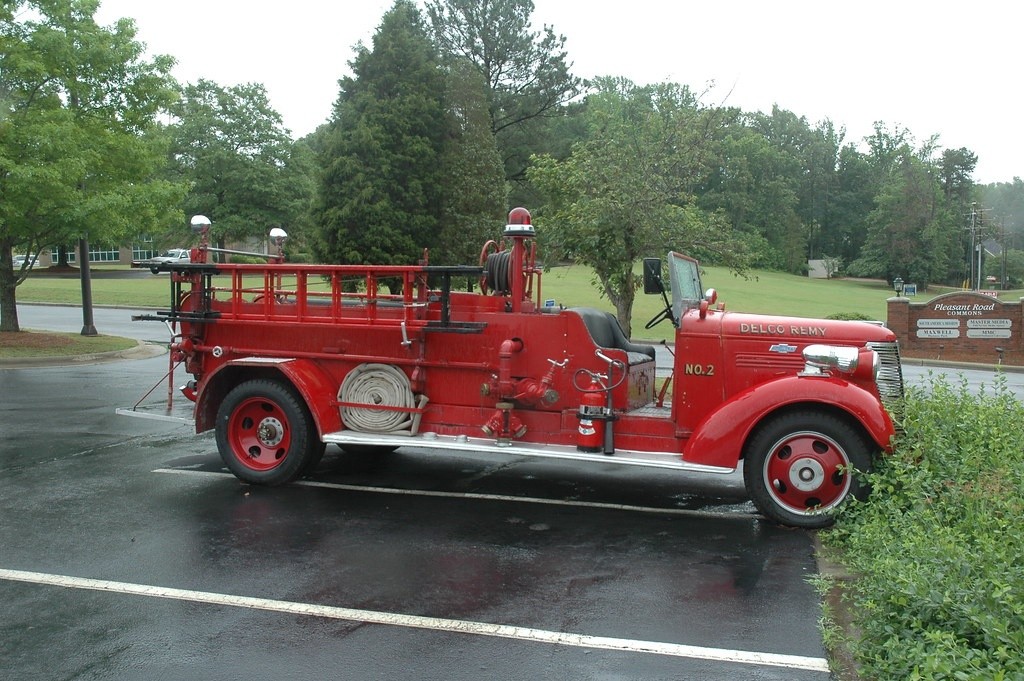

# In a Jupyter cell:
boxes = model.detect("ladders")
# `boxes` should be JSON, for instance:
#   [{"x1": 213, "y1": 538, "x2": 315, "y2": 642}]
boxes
[{"x1": 130, "y1": 261, "x2": 486, "y2": 334}]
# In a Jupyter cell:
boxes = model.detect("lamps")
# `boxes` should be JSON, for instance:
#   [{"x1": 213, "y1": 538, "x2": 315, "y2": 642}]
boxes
[{"x1": 893, "y1": 274, "x2": 904, "y2": 297}]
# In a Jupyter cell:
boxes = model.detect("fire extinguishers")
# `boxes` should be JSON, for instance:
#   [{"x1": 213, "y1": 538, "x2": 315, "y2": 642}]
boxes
[{"x1": 573, "y1": 359, "x2": 626, "y2": 454}]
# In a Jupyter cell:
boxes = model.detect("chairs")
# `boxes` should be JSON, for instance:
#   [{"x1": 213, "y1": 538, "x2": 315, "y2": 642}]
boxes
[{"x1": 564, "y1": 308, "x2": 656, "y2": 413}]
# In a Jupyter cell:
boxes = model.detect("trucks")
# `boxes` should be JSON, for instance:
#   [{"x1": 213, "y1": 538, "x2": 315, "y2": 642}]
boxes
[{"x1": 115, "y1": 207, "x2": 905, "y2": 530}]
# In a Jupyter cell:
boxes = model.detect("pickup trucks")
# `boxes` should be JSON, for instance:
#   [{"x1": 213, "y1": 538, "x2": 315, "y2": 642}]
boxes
[{"x1": 150, "y1": 249, "x2": 191, "y2": 275}]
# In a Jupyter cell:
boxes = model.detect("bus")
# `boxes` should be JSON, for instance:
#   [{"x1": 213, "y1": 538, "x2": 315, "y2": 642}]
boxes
[{"x1": 12, "y1": 255, "x2": 39, "y2": 267}]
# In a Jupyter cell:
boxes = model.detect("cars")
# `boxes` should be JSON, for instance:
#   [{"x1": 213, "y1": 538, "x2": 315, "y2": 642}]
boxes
[{"x1": 986, "y1": 276, "x2": 997, "y2": 282}]
